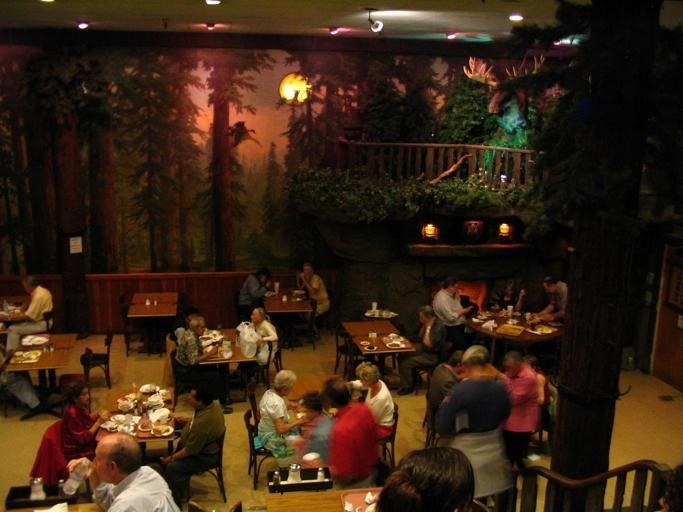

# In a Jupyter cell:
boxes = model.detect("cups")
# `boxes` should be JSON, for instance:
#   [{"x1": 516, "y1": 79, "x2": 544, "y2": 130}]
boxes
[
  {"x1": 525, "y1": 312, "x2": 531, "y2": 320},
  {"x1": 371, "y1": 303, "x2": 376, "y2": 311},
  {"x1": 2, "y1": 299, "x2": 27, "y2": 319},
  {"x1": 263, "y1": 281, "x2": 304, "y2": 303},
  {"x1": 506, "y1": 305, "x2": 513, "y2": 319}
]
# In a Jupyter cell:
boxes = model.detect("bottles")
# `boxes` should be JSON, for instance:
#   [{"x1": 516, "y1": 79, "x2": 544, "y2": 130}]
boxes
[
  {"x1": 56, "y1": 479, "x2": 67, "y2": 499},
  {"x1": 286, "y1": 463, "x2": 303, "y2": 483},
  {"x1": 144, "y1": 296, "x2": 157, "y2": 306},
  {"x1": 29, "y1": 477, "x2": 47, "y2": 499},
  {"x1": 272, "y1": 471, "x2": 280, "y2": 485},
  {"x1": 317, "y1": 467, "x2": 324, "y2": 482},
  {"x1": 199, "y1": 327, "x2": 240, "y2": 359},
  {"x1": 62, "y1": 459, "x2": 89, "y2": 495}
]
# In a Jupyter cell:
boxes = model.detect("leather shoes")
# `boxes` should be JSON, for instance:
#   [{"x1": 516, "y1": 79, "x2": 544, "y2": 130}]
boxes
[
  {"x1": 223, "y1": 399, "x2": 232, "y2": 413},
  {"x1": 397, "y1": 387, "x2": 413, "y2": 395}
]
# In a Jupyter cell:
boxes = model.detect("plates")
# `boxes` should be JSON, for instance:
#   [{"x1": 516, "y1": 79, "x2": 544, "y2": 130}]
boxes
[
  {"x1": 360, "y1": 330, "x2": 405, "y2": 351},
  {"x1": 497, "y1": 325, "x2": 524, "y2": 337},
  {"x1": 365, "y1": 502, "x2": 375, "y2": 512},
  {"x1": 107, "y1": 383, "x2": 175, "y2": 437},
  {"x1": 525, "y1": 324, "x2": 558, "y2": 335},
  {"x1": 14, "y1": 334, "x2": 47, "y2": 366}
]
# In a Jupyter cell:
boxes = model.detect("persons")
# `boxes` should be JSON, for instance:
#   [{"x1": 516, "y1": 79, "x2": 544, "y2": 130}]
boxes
[
  {"x1": 238, "y1": 266, "x2": 271, "y2": 319},
  {"x1": 64, "y1": 432, "x2": 181, "y2": 512},
  {"x1": 145, "y1": 387, "x2": 226, "y2": 505},
  {"x1": 173, "y1": 315, "x2": 234, "y2": 413},
  {"x1": 60, "y1": 382, "x2": 108, "y2": 461},
  {"x1": 295, "y1": 261, "x2": 330, "y2": 342},
  {"x1": 1, "y1": 273, "x2": 53, "y2": 354},
  {"x1": 231, "y1": 306, "x2": 278, "y2": 389},
  {"x1": 255, "y1": 275, "x2": 570, "y2": 511}
]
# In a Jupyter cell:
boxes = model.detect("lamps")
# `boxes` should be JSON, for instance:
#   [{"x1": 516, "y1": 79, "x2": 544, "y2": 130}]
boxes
[
  {"x1": 364, "y1": 6, "x2": 384, "y2": 33},
  {"x1": 497, "y1": 220, "x2": 514, "y2": 240},
  {"x1": 419, "y1": 220, "x2": 441, "y2": 241}
]
